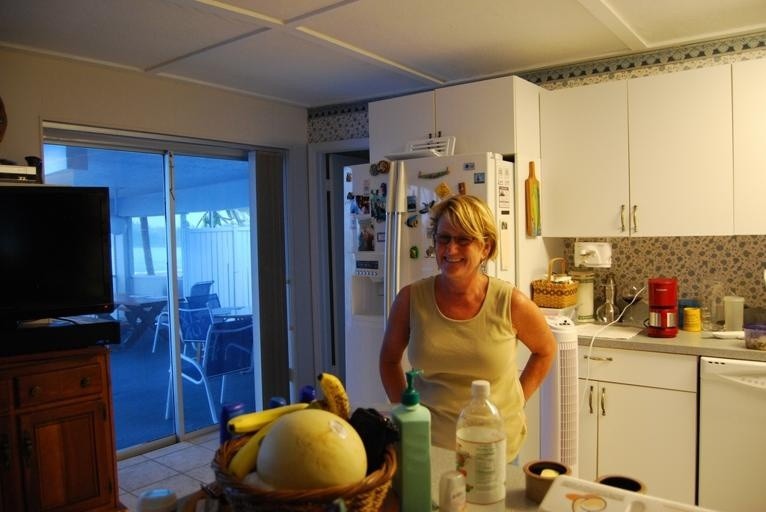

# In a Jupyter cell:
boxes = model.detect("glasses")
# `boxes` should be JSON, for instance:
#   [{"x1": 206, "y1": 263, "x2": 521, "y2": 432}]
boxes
[{"x1": 433, "y1": 233, "x2": 488, "y2": 246}]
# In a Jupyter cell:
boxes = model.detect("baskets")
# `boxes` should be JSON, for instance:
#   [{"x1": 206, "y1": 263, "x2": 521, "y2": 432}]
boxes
[
  {"x1": 530, "y1": 258, "x2": 580, "y2": 309},
  {"x1": 211, "y1": 432, "x2": 398, "y2": 511}
]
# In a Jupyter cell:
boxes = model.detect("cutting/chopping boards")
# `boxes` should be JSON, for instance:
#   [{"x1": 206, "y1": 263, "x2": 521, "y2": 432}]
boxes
[{"x1": 524, "y1": 161, "x2": 542, "y2": 238}]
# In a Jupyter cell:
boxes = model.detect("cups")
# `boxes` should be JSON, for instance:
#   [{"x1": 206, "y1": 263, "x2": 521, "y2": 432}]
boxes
[
  {"x1": 523, "y1": 460, "x2": 572, "y2": 503},
  {"x1": 597, "y1": 474, "x2": 649, "y2": 495},
  {"x1": 725, "y1": 296, "x2": 745, "y2": 331},
  {"x1": 684, "y1": 307, "x2": 702, "y2": 333}
]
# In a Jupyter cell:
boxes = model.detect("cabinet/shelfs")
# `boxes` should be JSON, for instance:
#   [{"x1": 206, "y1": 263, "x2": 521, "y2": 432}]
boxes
[
  {"x1": 1, "y1": 343, "x2": 132, "y2": 512},
  {"x1": 368, "y1": 75, "x2": 552, "y2": 163},
  {"x1": 536, "y1": 58, "x2": 765, "y2": 238},
  {"x1": 516, "y1": 340, "x2": 699, "y2": 512}
]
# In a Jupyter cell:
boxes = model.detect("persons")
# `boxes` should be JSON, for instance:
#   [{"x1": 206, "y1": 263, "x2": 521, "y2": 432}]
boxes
[{"x1": 377, "y1": 193, "x2": 561, "y2": 469}]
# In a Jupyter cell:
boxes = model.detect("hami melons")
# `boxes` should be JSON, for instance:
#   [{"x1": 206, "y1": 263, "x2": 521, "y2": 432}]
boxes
[{"x1": 256, "y1": 409, "x2": 368, "y2": 489}]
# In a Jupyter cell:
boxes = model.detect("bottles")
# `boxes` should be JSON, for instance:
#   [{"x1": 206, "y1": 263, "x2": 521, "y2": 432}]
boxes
[
  {"x1": 646, "y1": 278, "x2": 679, "y2": 338},
  {"x1": 604, "y1": 274, "x2": 618, "y2": 305},
  {"x1": 454, "y1": 379, "x2": 508, "y2": 506}
]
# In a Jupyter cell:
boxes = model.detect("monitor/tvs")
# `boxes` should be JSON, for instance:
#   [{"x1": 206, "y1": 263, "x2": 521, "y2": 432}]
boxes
[{"x1": 0, "y1": 182, "x2": 115, "y2": 330}]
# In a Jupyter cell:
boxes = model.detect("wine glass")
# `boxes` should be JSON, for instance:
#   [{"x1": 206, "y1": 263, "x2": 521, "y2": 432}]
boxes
[
  {"x1": 623, "y1": 285, "x2": 643, "y2": 324},
  {"x1": 594, "y1": 283, "x2": 619, "y2": 324}
]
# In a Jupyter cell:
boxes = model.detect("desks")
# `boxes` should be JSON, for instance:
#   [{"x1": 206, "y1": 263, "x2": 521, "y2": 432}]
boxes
[{"x1": 114, "y1": 295, "x2": 185, "y2": 349}]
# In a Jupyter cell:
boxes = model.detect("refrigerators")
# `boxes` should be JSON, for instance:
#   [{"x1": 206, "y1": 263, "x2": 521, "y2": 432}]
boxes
[{"x1": 340, "y1": 152, "x2": 566, "y2": 467}]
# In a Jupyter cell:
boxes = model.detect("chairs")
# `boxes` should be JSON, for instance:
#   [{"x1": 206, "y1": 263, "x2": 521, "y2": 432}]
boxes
[{"x1": 151, "y1": 281, "x2": 254, "y2": 424}]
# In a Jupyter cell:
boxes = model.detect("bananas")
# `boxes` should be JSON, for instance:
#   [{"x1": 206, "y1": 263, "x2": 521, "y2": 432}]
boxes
[{"x1": 227, "y1": 372, "x2": 350, "y2": 483}]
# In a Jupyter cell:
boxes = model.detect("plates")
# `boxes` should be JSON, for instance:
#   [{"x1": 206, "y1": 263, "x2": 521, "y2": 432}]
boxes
[{"x1": 714, "y1": 331, "x2": 745, "y2": 339}]
